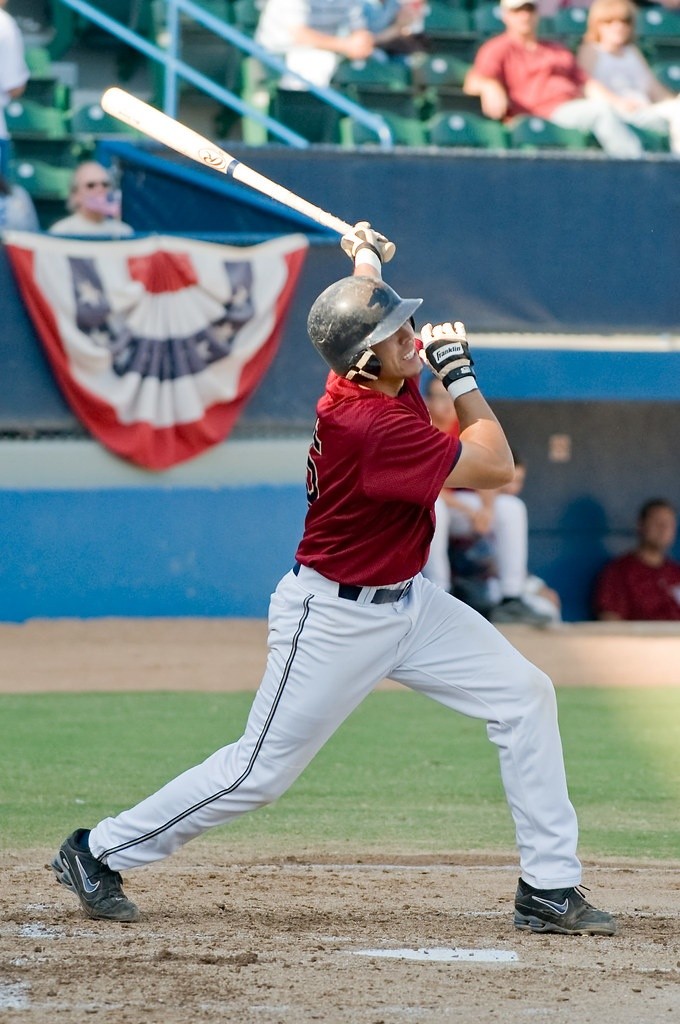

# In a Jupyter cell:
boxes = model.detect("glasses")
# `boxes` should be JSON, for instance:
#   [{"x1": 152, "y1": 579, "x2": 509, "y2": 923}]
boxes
[{"x1": 85, "y1": 181, "x2": 110, "y2": 188}]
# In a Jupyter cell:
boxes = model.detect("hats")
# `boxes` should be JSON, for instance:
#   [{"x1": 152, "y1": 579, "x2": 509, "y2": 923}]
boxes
[{"x1": 500, "y1": 0, "x2": 536, "y2": 10}]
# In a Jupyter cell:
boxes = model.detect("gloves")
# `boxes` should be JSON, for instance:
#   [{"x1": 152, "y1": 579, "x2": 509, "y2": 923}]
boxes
[
  {"x1": 419, "y1": 322, "x2": 479, "y2": 402},
  {"x1": 341, "y1": 222, "x2": 389, "y2": 276}
]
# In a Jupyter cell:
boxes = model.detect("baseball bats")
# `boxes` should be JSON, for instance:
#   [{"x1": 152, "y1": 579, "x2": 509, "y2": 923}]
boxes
[{"x1": 100, "y1": 86, "x2": 397, "y2": 265}]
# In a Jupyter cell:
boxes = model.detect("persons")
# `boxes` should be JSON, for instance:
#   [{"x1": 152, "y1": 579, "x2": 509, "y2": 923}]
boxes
[
  {"x1": 53, "y1": 222, "x2": 617, "y2": 936},
  {"x1": 0, "y1": 0, "x2": 40, "y2": 235},
  {"x1": 420, "y1": 372, "x2": 563, "y2": 625},
  {"x1": 593, "y1": 501, "x2": 680, "y2": 621},
  {"x1": 49, "y1": 163, "x2": 132, "y2": 238},
  {"x1": 254, "y1": 0, "x2": 680, "y2": 154}
]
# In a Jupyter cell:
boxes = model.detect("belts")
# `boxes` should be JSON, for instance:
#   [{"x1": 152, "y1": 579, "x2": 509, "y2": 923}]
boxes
[{"x1": 293, "y1": 561, "x2": 414, "y2": 604}]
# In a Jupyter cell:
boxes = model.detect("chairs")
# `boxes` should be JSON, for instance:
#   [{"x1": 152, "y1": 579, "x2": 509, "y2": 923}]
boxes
[{"x1": 0, "y1": 0, "x2": 680, "y2": 231}]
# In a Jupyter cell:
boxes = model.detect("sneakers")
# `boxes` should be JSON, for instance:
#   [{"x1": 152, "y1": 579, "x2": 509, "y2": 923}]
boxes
[
  {"x1": 514, "y1": 877, "x2": 616, "y2": 934},
  {"x1": 51, "y1": 828, "x2": 140, "y2": 921}
]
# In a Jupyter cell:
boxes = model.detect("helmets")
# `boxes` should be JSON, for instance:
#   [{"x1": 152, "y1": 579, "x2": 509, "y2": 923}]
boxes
[{"x1": 307, "y1": 276, "x2": 424, "y2": 382}]
